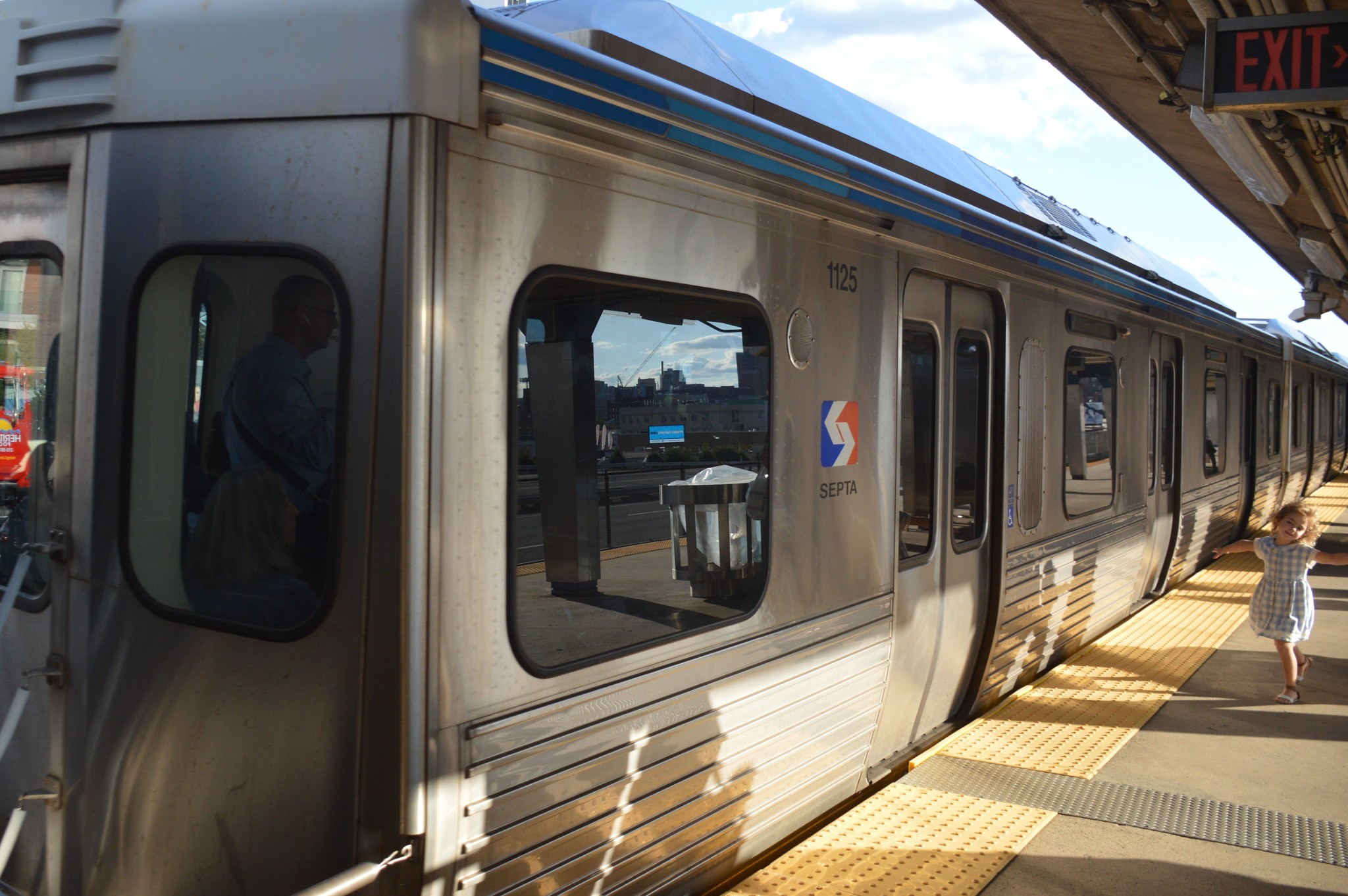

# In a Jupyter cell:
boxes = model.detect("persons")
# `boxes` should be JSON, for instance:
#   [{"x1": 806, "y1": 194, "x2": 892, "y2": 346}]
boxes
[
  {"x1": 1204, "y1": 425, "x2": 1218, "y2": 472},
  {"x1": 1212, "y1": 500, "x2": 1348, "y2": 704}
]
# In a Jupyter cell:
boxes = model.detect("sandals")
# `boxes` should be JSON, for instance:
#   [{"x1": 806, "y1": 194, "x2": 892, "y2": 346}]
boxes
[
  {"x1": 1274, "y1": 685, "x2": 1300, "y2": 704},
  {"x1": 1296, "y1": 656, "x2": 1313, "y2": 683}
]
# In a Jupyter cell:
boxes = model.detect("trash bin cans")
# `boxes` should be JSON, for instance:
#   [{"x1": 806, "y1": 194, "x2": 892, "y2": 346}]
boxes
[{"x1": 659, "y1": 482, "x2": 760, "y2": 599}]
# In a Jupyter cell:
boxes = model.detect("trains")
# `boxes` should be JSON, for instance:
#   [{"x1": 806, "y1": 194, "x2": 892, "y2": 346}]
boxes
[{"x1": 0, "y1": 0, "x2": 1348, "y2": 896}]
